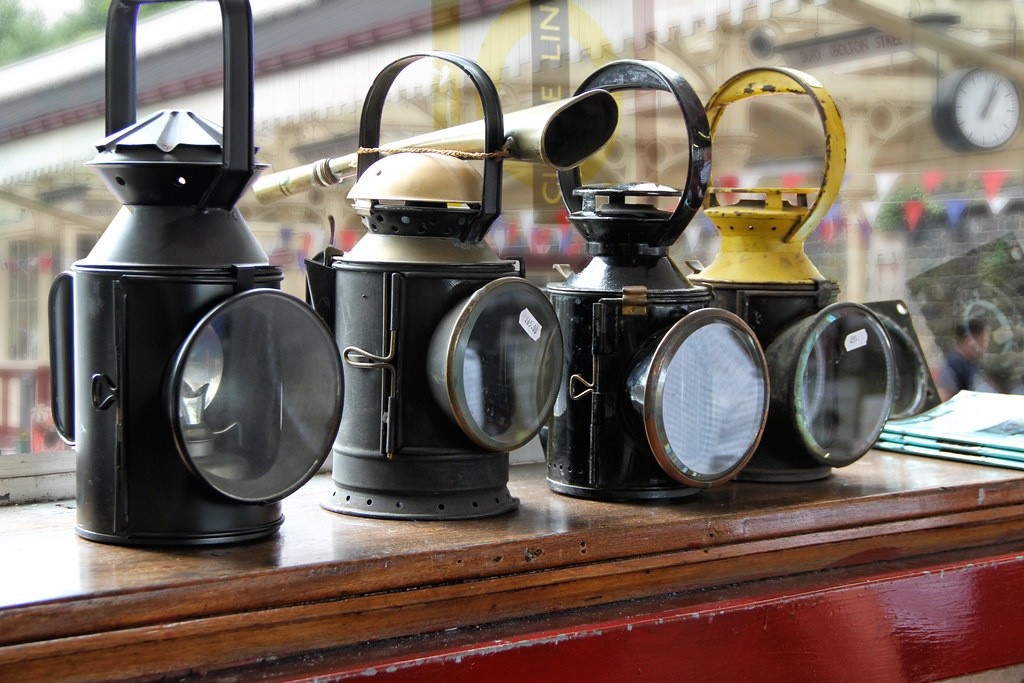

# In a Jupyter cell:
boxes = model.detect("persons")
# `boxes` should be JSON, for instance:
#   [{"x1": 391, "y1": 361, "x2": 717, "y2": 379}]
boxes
[{"x1": 942, "y1": 318, "x2": 1005, "y2": 400}]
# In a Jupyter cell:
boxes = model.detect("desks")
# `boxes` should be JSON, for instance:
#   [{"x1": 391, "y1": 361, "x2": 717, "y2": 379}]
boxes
[{"x1": 0, "y1": 444, "x2": 1024, "y2": 683}]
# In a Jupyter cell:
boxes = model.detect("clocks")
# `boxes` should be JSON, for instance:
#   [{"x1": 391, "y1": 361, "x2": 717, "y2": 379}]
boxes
[{"x1": 933, "y1": 66, "x2": 1021, "y2": 154}]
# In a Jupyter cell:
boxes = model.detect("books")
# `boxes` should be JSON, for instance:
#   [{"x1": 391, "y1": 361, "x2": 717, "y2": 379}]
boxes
[{"x1": 874, "y1": 390, "x2": 1024, "y2": 471}]
